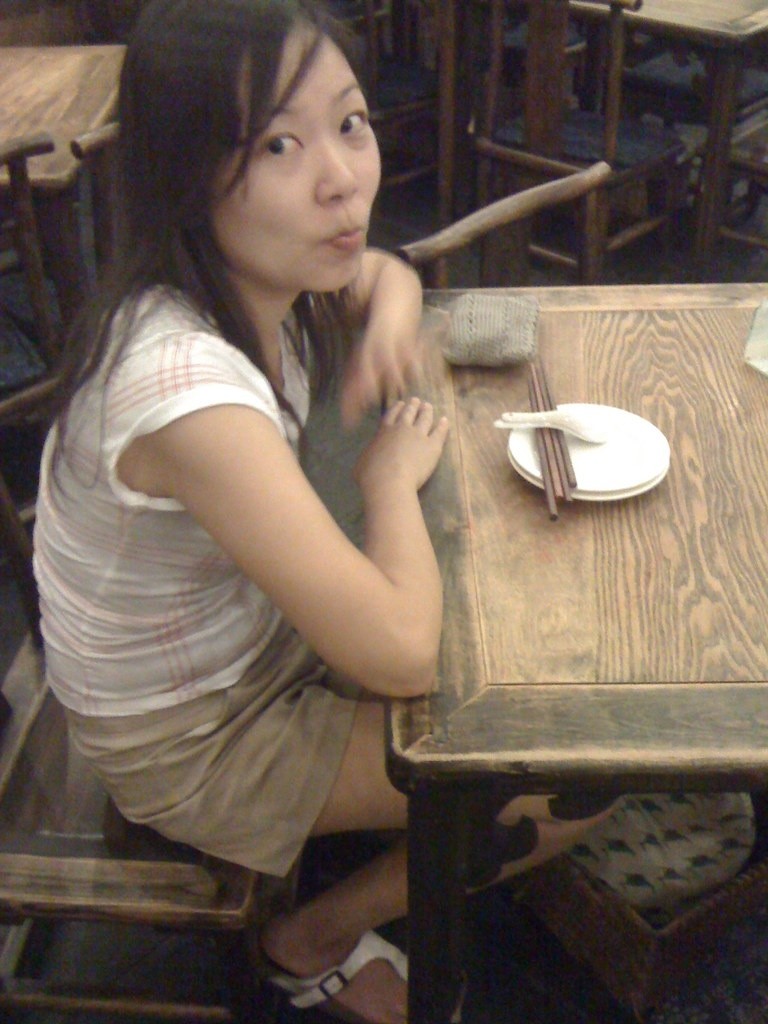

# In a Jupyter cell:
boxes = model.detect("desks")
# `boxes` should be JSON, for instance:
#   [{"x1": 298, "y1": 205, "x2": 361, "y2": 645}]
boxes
[
  {"x1": 387, "y1": 284, "x2": 768, "y2": 1024},
  {"x1": 569, "y1": 0, "x2": 768, "y2": 260}
]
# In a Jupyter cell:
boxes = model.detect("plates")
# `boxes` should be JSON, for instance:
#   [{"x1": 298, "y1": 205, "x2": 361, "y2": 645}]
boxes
[{"x1": 506, "y1": 402, "x2": 670, "y2": 502}]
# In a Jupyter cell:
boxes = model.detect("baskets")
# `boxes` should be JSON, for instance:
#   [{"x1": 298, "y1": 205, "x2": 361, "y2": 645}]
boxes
[{"x1": 521, "y1": 852, "x2": 768, "y2": 1024}]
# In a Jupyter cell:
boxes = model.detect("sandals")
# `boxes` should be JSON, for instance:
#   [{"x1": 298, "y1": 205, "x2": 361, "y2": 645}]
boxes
[{"x1": 254, "y1": 928, "x2": 408, "y2": 1024}]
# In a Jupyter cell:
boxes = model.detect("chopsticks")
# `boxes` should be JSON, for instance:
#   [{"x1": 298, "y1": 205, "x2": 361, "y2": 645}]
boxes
[{"x1": 524, "y1": 356, "x2": 579, "y2": 521}]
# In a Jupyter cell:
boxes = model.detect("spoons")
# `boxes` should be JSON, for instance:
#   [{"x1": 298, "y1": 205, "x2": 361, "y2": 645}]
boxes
[{"x1": 493, "y1": 413, "x2": 610, "y2": 443}]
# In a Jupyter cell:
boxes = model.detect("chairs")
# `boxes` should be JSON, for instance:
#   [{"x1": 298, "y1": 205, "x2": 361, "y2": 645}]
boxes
[{"x1": 0, "y1": 0, "x2": 694, "y2": 1024}]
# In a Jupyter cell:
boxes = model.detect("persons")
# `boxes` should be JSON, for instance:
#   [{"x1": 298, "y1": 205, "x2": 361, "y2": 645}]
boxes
[{"x1": 31, "y1": 0, "x2": 449, "y2": 1024}]
